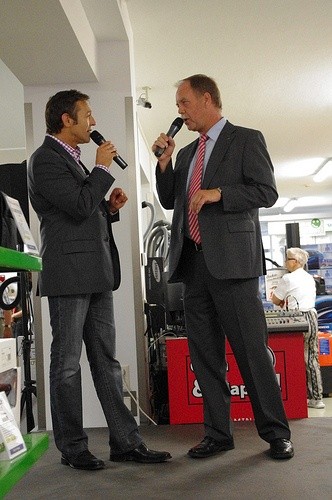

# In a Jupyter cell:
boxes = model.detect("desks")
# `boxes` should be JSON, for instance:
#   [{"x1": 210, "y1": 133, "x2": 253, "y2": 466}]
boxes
[{"x1": 164, "y1": 332, "x2": 308, "y2": 425}]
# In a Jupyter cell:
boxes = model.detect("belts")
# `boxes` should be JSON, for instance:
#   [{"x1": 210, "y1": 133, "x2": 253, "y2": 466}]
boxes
[{"x1": 183, "y1": 236, "x2": 202, "y2": 252}]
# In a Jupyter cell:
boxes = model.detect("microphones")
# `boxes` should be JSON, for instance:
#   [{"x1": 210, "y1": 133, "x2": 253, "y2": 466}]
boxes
[
  {"x1": 155, "y1": 117, "x2": 184, "y2": 157},
  {"x1": 90, "y1": 130, "x2": 128, "y2": 170}
]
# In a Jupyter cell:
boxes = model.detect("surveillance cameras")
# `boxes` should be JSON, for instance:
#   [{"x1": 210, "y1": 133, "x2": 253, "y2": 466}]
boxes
[{"x1": 138, "y1": 98, "x2": 152, "y2": 108}]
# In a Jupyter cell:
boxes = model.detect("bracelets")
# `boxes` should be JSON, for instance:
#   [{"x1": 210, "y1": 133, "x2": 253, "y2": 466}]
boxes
[{"x1": 217, "y1": 186, "x2": 223, "y2": 192}]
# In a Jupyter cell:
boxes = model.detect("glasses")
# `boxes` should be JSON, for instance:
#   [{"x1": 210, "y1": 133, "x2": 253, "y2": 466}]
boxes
[{"x1": 286, "y1": 258, "x2": 294, "y2": 262}]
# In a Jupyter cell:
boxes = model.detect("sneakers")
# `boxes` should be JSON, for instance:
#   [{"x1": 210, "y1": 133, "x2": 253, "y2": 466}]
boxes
[{"x1": 307, "y1": 399, "x2": 325, "y2": 408}]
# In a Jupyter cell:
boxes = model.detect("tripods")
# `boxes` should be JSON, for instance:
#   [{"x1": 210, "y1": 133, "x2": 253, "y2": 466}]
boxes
[{"x1": 18, "y1": 270, "x2": 38, "y2": 435}]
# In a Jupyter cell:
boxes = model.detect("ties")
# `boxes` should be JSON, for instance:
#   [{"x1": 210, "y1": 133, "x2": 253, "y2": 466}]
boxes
[
  {"x1": 188, "y1": 133, "x2": 210, "y2": 245},
  {"x1": 79, "y1": 160, "x2": 91, "y2": 175}
]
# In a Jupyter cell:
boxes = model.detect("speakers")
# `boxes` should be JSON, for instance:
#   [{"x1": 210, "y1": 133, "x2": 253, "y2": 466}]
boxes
[{"x1": 0, "y1": 163, "x2": 30, "y2": 253}]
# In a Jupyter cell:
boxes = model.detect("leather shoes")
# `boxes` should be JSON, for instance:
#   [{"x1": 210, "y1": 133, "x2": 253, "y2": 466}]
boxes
[
  {"x1": 270, "y1": 438, "x2": 294, "y2": 459},
  {"x1": 110, "y1": 441, "x2": 172, "y2": 463},
  {"x1": 188, "y1": 436, "x2": 235, "y2": 458},
  {"x1": 61, "y1": 448, "x2": 106, "y2": 469}
]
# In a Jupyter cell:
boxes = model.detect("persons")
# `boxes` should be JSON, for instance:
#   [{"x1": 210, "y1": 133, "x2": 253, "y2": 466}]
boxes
[
  {"x1": 270, "y1": 248, "x2": 326, "y2": 408},
  {"x1": 27, "y1": 90, "x2": 173, "y2": 470},
  {"x1": 151, "y1": 74, "x2": 295, "y2": 459}
]
